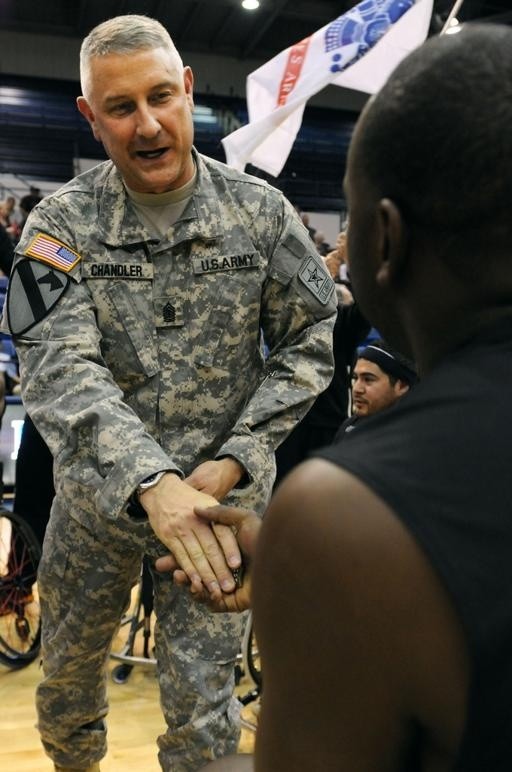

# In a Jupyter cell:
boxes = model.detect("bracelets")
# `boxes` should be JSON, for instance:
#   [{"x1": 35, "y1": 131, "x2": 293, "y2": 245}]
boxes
[{"x1": 137, "y1": 469, "x2": 167, "y2": 497}]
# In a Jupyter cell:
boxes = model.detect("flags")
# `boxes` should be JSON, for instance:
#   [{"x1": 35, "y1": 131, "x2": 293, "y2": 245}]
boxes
[{"x1": 221, "y1": 1, "x2": 434, "y2": 178}]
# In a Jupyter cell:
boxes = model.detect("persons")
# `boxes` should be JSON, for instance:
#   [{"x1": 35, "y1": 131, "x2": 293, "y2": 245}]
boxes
[
  {"x1": 9, "y1": 16, "x2": 341, "y2": 771},
  {"x1": 195, "y1": 20, "x2": 508, "y2": 770}
]
